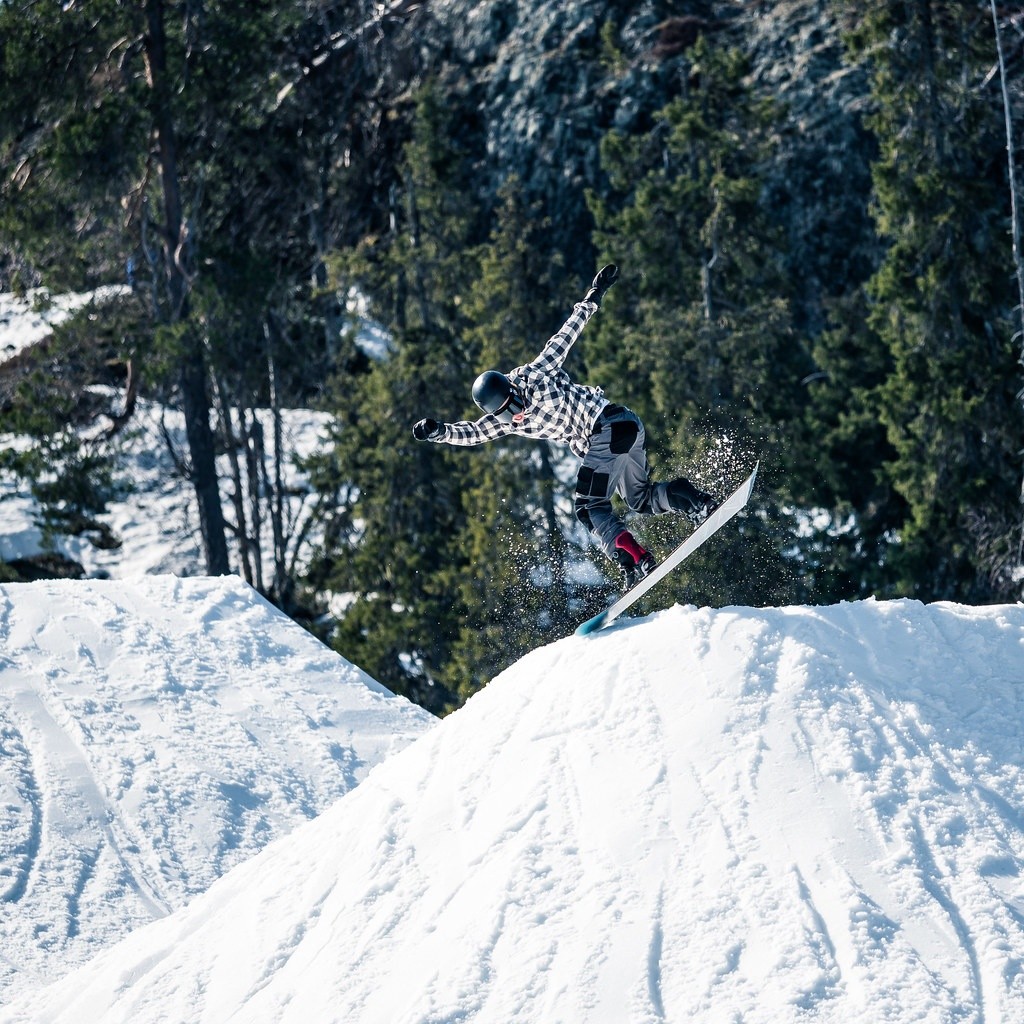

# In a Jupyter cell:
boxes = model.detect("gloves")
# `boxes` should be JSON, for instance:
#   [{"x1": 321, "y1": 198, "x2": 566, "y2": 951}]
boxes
[
  {"x1": 412, "y1": 418, "x2": 441, "y2": 441},
  {"x1": 584, "y1": 264, "x2": 618, "y2": 310}
]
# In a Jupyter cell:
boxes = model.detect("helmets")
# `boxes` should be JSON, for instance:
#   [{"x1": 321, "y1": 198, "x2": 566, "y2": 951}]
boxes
[{"x1": 472, "y1": 371, "x2": 510, "y2": 415}]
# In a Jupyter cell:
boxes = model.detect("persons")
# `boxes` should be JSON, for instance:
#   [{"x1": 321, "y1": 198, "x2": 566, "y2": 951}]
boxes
[{"x1": 412, "y1": 264, "x2": 717, "y2": 593}]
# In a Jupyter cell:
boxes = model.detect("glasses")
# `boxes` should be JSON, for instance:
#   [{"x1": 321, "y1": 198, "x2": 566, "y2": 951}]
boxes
[{"x1": 493, "y1": 393, "x2": 525, "y2": 424}]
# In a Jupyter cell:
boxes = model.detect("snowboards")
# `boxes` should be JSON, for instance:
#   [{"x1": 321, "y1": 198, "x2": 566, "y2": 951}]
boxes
[{"x1": 574, "y1": 458, "x2": 761, "y2": 638}]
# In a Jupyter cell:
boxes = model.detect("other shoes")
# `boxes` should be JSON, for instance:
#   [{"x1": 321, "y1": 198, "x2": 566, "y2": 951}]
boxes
[
  {"x1": 606, "y1": 531, "x2": 657, "y2": 594},
  {"x1": 666, "y1": 477, "x2": 717, "y2": 530}
]
